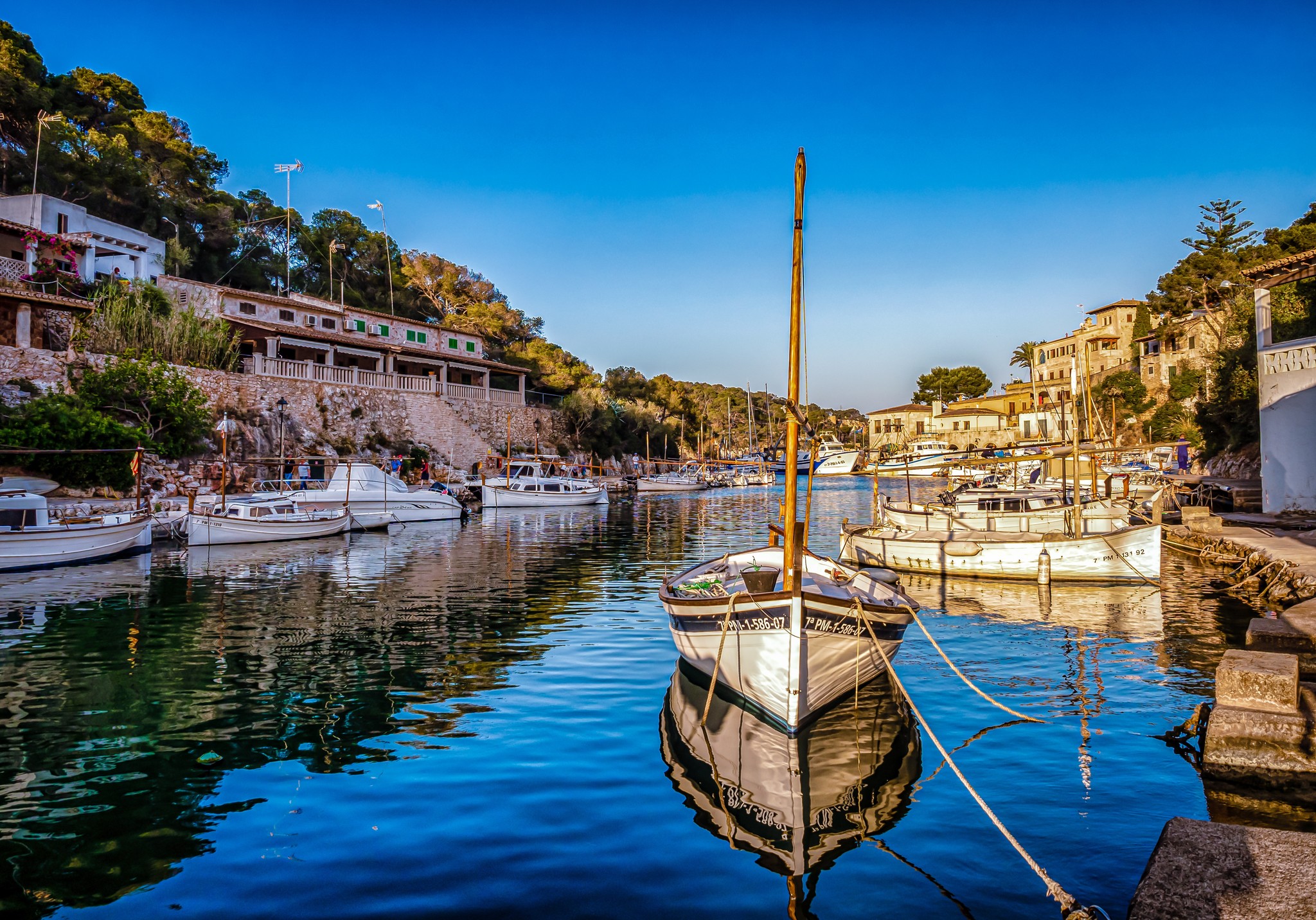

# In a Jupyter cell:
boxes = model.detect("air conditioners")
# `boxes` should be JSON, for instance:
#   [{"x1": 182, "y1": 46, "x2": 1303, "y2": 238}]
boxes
[
  {"x1": 344, "y1": 320, "x2": 357, "y2": 331},
  {"x1": 369, "y1": 325, "x2": 381, "y2": 335}
]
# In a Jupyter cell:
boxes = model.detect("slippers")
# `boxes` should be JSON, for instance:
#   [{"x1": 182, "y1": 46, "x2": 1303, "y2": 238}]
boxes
[{"x1": 418, "y1": 487, "x2": 423, "y2": 489}]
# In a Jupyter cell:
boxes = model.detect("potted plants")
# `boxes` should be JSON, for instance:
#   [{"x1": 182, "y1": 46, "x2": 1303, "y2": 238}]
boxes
[{"x1": 305, "y1": 315, "x2": 318, "y2": 326}]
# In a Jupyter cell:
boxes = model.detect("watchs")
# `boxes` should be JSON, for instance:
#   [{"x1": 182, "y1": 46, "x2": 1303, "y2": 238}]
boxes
[{"x1": 291, "y1": 472, "x2": 292, "y2": 474}]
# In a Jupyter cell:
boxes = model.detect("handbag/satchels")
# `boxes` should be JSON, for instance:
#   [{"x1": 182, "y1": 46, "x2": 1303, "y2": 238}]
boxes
[
  {"x1": 558, "y1": 462, "x2": 563, "y2": 468},
  {"x1": 638, "y1": 464, "x2": 639, "y2": 467}
]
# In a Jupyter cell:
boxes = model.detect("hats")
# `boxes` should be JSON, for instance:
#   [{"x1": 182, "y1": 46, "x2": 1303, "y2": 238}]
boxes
[
  {"x1": 584, "y1": 459, "x2": 587, "y2": 462},
  {"x1": 398, "y1": 455, "x2": 402, "y2": 458}
]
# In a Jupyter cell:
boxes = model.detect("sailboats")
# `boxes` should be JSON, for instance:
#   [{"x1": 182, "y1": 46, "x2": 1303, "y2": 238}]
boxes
[
  {"x1": 635, "y1": 381, "x2": 776, "y2": 491},
  {"x1": 655, "y1": 147, "x2": 923, "y2": 736},
  {"x1": 479, "y1": 412, "x2": 611, "y2": 508}
]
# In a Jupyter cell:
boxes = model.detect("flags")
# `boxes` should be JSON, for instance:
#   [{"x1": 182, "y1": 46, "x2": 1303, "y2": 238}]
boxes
[
  {"x1": 849, "y1": 425, "x2": 854, "y2": 437},
  {"x1": 129, "y1": 447, "x2": 139, "y2": 477},
  {"x1": 855, "y1": 427, "x2": 863, "y2": 433}
]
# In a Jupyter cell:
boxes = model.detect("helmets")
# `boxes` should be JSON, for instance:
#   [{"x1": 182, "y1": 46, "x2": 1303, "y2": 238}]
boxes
[{"x1": 634, "y1": 453, "x2": 637, "y2": 455}]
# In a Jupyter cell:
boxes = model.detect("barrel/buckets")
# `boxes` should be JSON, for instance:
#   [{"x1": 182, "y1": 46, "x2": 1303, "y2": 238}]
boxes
[{"x1": 739, "y1": 566, "x2": 782, "y2": 593}]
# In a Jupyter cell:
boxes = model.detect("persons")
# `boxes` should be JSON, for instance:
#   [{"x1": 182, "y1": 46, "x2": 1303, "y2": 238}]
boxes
[
  {"x1": 297, "y1": 456, "x2": 311, "y2": 490},
  {"x1": 534, "y1": 457, "x2": 543, "y2": 472},
  {"x1": 580, "y1": 459, "x2": 587, "y2": 479},
  {"x1": 282, "y1": 454, "x2": 295, "y2": 491},
  {"x1": 389, "y1": 455, "x2": 403, "y2": 479},
  {"x1": 558, "y1": 457, "x2": 566, "y2": 477},
  {"x1": 632, "y1": 453, "x2": 639, "y2": 475},
  {"x1": 549, "y1": 459, "x2": 556, "y2": 477},
  {"x1": 1172, "y1": 433, "x2": 1194, "y2": 475},
  {"x1": 572, "y1": 464, "x2": 582, "y2": 479},
  {"x1": 418, "y1": 457, "x2": 431, "y2": 489}
]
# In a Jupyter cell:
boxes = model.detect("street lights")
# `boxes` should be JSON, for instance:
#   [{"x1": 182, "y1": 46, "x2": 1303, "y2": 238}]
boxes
[
  {"x1": 533, "y1": 417, "x2": 542, "y2": 463},
  {"x1": 275, "y1": 396, "x2": 288, "y2": 490}
]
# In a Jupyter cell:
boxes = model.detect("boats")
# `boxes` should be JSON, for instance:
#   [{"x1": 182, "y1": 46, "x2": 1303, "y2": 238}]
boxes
[
  {"x1": 839, "y1": 348, "x2": 1169, "y2": 587},
  {"x1": 863, "y1": 432, "x2": 971, "y2": 474},
  {"x1": 765, "y1": 428, "x2": 862, "y2": 475},
  {"x1": 0, "y1": 455, "x2": 471, "y2": 573},
  {"x1": 654, "y1": 653, "x2": 928, "y2": 920}
]
